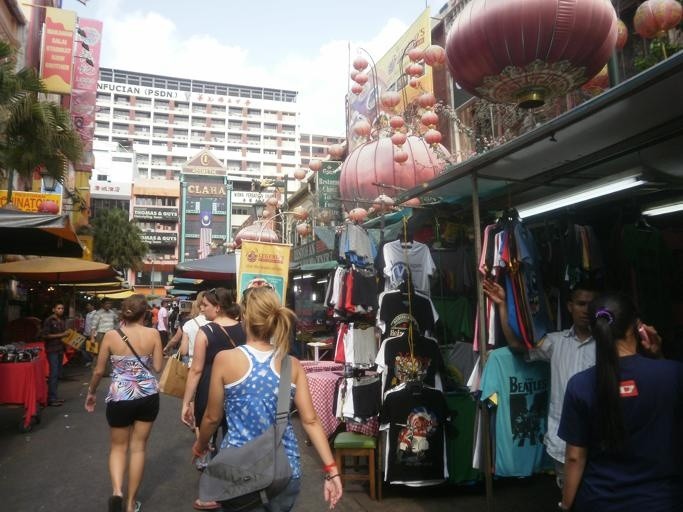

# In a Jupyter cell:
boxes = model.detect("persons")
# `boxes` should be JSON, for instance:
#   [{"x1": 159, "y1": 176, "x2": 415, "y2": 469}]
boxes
[
  {"x1": 193, "y1": 282, "x2": 345, "y2": 511},
  {"x1": 161, "y1": 282, "x2": 246, "y2": 508},
  {"x1": 68, "y1": 291, "x2": 183, "y2": 377},
  {"x1": 82, "y1": 294, "x2": 167, "y2": 512},
  {"x1": 553, "y1": 291, "x2": 682, "y2": 512},
  {"x1": 481, "y1": 275, "x2": 597, "y2": 493},
  {"x1": 39, "y1": 302, "x2": 72, "y2": 408}
]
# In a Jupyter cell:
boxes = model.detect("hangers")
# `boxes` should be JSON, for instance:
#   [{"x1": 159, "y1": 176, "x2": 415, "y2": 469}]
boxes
[{"x1": 407, "y1": 372, "x2": 426, "y2": 393}]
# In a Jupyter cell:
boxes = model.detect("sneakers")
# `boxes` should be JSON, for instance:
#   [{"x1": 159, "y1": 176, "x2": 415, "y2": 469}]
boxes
[{"x1": 193, "y1": 497, "x2": 222, "y2": 510}]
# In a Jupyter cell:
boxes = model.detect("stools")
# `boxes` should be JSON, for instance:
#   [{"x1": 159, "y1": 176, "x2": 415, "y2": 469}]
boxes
[{"x1": 333, "y1": 431, "x2": 376, "y2": 498}]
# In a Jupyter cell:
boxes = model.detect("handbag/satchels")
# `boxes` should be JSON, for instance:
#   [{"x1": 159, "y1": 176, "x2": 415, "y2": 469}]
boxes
[
  {"x1": 157, "y1": 348, "x2": 190, "y2": 399},
  {"x1": 198, "y1": 424, "x2": 294, "y2": 512}
]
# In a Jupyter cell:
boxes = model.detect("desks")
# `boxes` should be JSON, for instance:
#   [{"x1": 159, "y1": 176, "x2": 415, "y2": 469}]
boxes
[
  {"x1": 0, "y1": 340, "x2": 48, "y2": 431},
  {"x1": 297, "y1": 356, "x2": 380, "y2": 435}
]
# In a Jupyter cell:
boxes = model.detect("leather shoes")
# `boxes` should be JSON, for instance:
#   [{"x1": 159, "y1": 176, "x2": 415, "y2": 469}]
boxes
[
  {"x1": 56, "y1": 398, "x2": 65, "y2": 402},
  {"x1": 49, "y1": 402, "x2": 63, "y2": 406}
]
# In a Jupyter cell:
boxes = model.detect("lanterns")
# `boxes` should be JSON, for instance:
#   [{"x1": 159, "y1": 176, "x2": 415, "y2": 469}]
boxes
[
  {"x1": 629, "y1": 1, "x2": 683, "y2": 43},
  {"x1": 611, "y1": 16, "x2": 631, "y2": 53},
  {"x1": 443, "y1": 1, "x2": 622, "y2": 113},
  {"x1": 334, "y1": 28, "x2": 455, "y2": 225},
  {"x1": 235, "y1": 144, "x2": 348, "y2": 250}
]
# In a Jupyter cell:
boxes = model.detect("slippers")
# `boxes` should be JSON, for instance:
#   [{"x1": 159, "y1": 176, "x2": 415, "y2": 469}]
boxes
[
  {"x1": 134, "y1": 501, "x2": 141, "y2": 512},
  {"x1": 108, "y1": 495, "x2": 123, "y2": 512}
]
